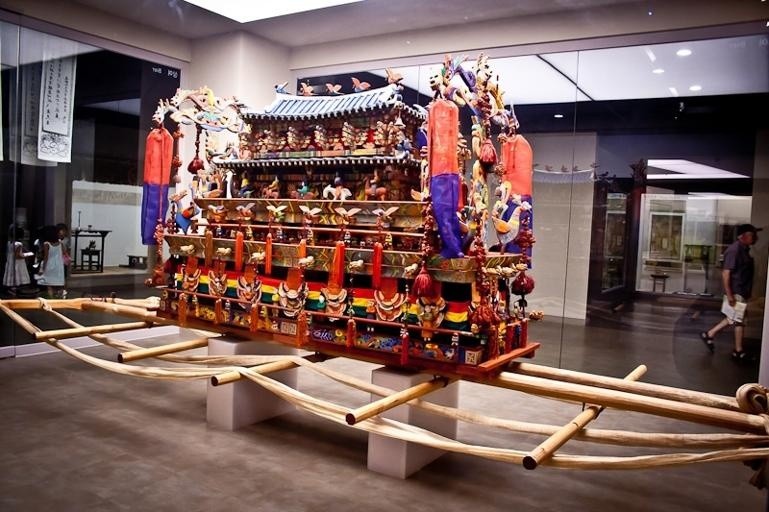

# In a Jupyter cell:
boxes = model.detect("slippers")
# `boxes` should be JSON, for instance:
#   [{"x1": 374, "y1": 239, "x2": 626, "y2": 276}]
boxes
[{"x1": 700, "y1": 331, "x2": 715, "y2": 355}]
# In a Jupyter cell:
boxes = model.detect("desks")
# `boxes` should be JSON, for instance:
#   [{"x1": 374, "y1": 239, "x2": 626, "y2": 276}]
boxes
[{"x1": 71, "y1": 228, "x2": 113, "y2": 273}]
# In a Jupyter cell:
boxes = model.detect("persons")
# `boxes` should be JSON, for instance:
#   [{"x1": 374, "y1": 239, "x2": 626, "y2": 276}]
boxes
[
  {"x1": 2, "y1": 223, "x2": 71, "y2": 299},
  {"x1": 700, "y1": 224, "x2": 762, "y2": 362}
]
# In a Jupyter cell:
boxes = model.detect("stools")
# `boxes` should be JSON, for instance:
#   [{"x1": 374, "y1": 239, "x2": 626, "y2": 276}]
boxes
[{"x1": 118, "y1": 254, "x2": 149, "y2": 269}]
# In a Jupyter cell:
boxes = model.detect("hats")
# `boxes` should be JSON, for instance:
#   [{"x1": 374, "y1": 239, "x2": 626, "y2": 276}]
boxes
[{"x1": 736, "y1": 223, "x2": 763, "y2": 235}]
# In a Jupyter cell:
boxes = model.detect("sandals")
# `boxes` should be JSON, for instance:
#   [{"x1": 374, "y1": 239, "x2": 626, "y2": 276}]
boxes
[{"x1": 730, "y1": 350, "x2": 753, "y2": 362}]
